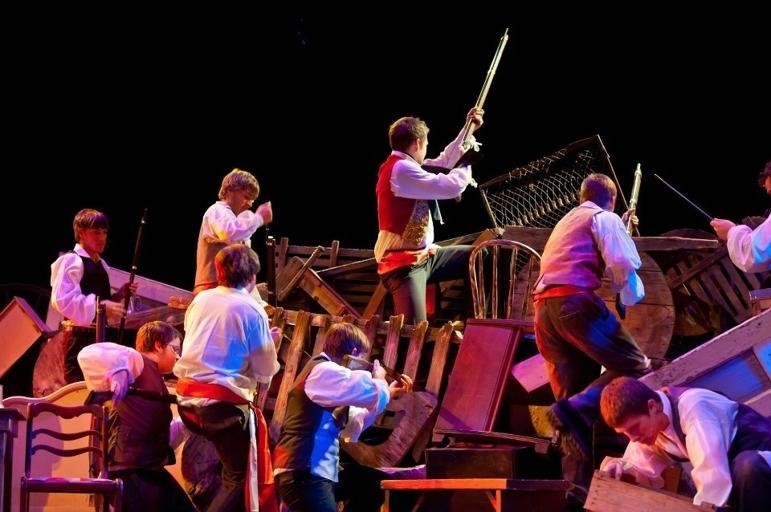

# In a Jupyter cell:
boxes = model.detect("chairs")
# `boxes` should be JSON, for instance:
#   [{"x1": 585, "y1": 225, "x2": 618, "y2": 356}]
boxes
[{"x1": 19, "y1": 402, "x2": 123, "y2": 512}]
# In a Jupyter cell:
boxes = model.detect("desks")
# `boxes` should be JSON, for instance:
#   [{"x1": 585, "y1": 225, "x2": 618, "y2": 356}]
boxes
[{"x1": 379, "y1": 479, "x2": 569, "y2": 512}]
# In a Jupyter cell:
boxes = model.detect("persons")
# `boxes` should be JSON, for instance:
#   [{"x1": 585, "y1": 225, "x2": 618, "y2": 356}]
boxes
[
  {"x1": 50, "y1": 207, "x2": 139, "y2": 385},
  {"x1": 710, "y1": 163, "x2": 771, "y2": 275},
  {"x1": 274, "y1": 320, "x2": 415, "y2": 510},
  {"x1": 529, "y1": 173, "x2": 653, "y2": 468},
  {"x1": 599, "y1": 375, "x2": 770, "y2": 512},
  {"x1": 77, "y1": 321, "x2": 197, "y2": 512},
  {"x1": 192, "y1": 167, "x2": 273, "y2": 309},
  {"x1": 170, "y1": 244, "x2": 284, "y2": 511},
  {"x1": 376, "y1": 108, "x2": 489, "y2": 328}
]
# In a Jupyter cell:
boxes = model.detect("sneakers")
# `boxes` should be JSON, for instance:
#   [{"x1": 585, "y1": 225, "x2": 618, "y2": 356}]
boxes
[{"x1": 546, "y1": 402, "x2": 592, "y2": 470}]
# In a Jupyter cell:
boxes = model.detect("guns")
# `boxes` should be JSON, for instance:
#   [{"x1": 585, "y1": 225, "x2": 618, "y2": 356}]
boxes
[
  {"x1": 616, "y1": 163, "x2": 642, "y2": 319},
  {"x1": 118, "y1": 210, "x2": 147, "y2": 344},
  {"x1": 458, "y1": 27, "x2": 510, "y2": 172},
  {"x1": 339, "y1": 354, "x2": 406, "y2": 382},
  {"x1": 265, "y1": 224, "x2": 277, "y2": 310},
  {"x1": 84, "y1": 390, "x2": 178, "y2": 408}
]
